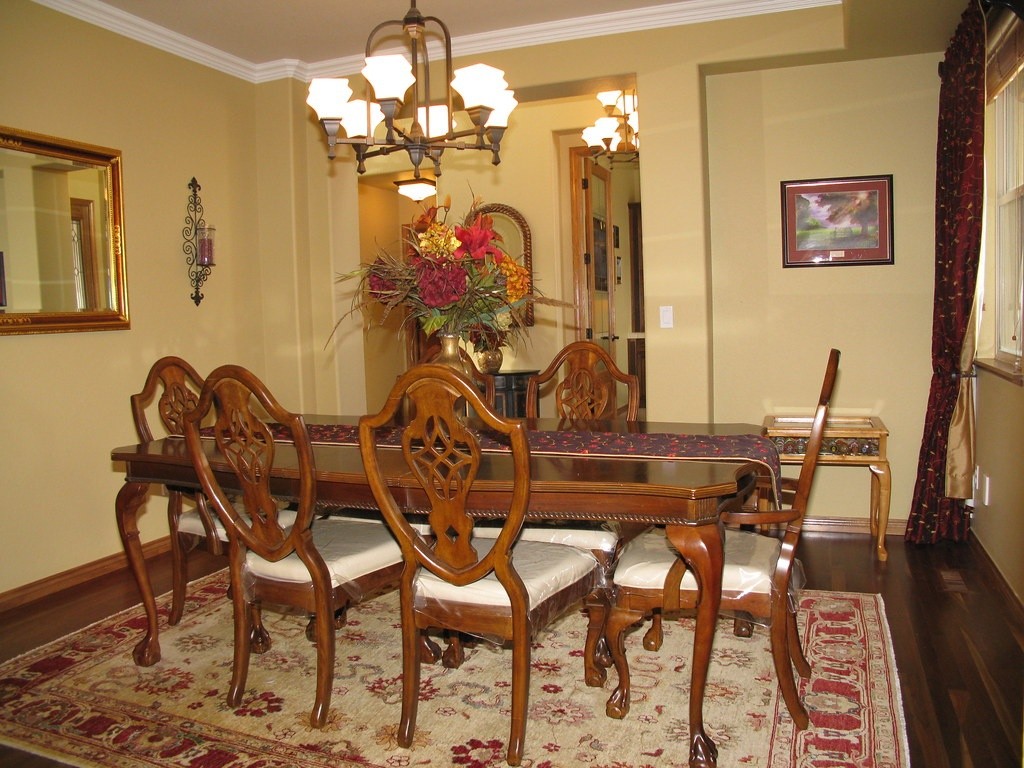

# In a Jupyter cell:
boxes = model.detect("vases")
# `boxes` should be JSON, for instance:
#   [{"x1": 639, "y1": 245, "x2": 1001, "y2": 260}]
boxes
[
  {"x1": 476, "y1": 347, "x2": 503, "y2": 373},
  {"x1": 431, "y1": 333, "x2": 473, "y2": 441}
]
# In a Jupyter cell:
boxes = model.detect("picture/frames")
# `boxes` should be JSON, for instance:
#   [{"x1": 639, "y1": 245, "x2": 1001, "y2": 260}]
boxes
[
  {"x1": 585, "y1": 212, "x2": 616, "y2": 295},
  {"x1": 780, "y1": 174, "x2": 895, "y2": 268}
]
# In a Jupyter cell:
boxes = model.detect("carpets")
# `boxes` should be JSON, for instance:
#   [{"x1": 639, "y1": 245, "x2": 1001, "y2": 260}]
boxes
[{"x1": 0, "y1": 567, "x2": 912, "y2": 768}]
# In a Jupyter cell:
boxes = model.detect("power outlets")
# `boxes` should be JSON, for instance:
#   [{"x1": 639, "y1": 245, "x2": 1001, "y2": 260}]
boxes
[
  {"x1": 974, "y1": 464, "x2": 980, "y2": 490},
  {"x1": 982, "y1": 474, "x2": 989, "y2": 506}
]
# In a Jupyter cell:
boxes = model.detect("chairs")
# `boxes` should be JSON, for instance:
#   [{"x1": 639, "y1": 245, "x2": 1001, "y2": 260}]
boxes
[
  {"x1": 182, "y1": 365, "x2": 442, "y2": 729},
  {"x1": 130, "y1": 355, "x2": 347, "y2": 654},
  {"x1": 604, "y1": 348, "x2": 841, "y2": 729},
  {"x1": 473, "y1": 341, "x2": 642, "y2": 669},
  {"x1": 359, "y1": 362, "x2": 609, "y2": 768}
]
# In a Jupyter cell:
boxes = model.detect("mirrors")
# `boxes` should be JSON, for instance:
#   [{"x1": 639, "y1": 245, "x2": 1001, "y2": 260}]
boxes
[{"x1": 0, "y1": 125, "x2": 131, "y2": 336}]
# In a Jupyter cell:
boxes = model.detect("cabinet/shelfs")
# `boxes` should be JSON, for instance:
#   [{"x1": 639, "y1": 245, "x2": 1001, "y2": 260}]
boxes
[{"x1": 468, "y1": 369, "x2": 541, "y2": 419}]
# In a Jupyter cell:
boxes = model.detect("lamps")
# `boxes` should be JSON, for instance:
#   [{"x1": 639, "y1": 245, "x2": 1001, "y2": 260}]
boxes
[
  {"x1": 306, "y1": 0, "x2": 519, "y2": 180},
  {"x1": 393, "y1": 178, "x2": 436, "y2": 205},
  {"x1": 182, "y1": 176, "x2": 217, "y2": 307},
  {"x1": 581, "y1": 89, "x2": 640, "y2": 171}
]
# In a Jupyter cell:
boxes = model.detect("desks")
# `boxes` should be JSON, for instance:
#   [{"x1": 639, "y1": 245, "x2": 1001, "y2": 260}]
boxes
[
  {"x1": 111, "y1": 413, "x2": 782, "y2": 767},
  {"x1": 763, "y1": 413, "x2": 892, "y2": 562}
]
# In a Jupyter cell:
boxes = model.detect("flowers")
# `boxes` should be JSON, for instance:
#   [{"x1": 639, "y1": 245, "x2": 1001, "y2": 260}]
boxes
[
  {"x1": 323, "y1": 177, "x2": 581, "y2": 365},
  {"x1": 469, "y1": 313, "x2": 514, "y2": 353}
]
